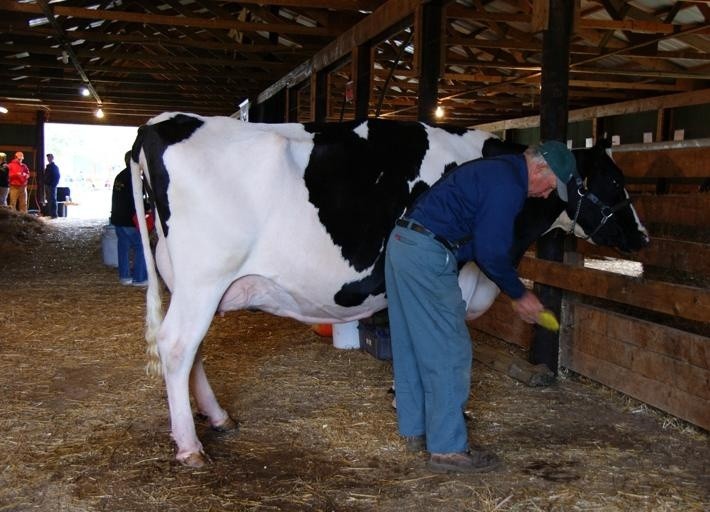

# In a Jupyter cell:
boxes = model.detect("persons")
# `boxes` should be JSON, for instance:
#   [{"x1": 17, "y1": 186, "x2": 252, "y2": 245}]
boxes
[
  {"x1": 0, "y1": 151, "x2": 11, "y2": 207},
  {"x1": 8, "y1": 150, "x2": 31, "y2": 213},
  {"x1": 383, "y1": 137, "x2": 579, "y2": 475},
  {"x1": 41, "y1": 154, "x2": 60, "y2": 219},
  {"x1": 110, "y1": 149, "x2": 149, "y2": 287}
]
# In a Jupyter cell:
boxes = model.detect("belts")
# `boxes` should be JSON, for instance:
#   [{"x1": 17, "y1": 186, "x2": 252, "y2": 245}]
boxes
[{"x1": 394, "y1": 219, "x2": 455, "y2": 253}]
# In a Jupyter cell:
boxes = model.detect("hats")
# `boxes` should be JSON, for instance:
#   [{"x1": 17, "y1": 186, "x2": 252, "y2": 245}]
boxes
[
  {"x1": 16, "y1": 152, "x2": 23, "y2": 159},
  {"x1": 540, "y1": 141, "x2": 575, "y2": 202}
]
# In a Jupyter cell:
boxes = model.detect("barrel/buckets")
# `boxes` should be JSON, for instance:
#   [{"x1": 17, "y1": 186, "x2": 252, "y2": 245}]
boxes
[
  {"x1": 332, "y1": 319, "x2": 362, "y2": 350},
  {"x1": 101, "y1": 224, "x2": 120, "y2": 269}
]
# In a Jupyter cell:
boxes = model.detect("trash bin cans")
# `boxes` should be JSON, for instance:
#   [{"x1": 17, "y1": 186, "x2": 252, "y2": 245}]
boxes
[{"x1": 57, "y1": 187, "x2": 70, "y2": 217}]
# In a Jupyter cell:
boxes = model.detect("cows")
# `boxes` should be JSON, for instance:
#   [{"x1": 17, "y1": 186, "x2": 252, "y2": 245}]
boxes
[{"x1": 129, "y1": 108, "x2": 651, "y2": 471}]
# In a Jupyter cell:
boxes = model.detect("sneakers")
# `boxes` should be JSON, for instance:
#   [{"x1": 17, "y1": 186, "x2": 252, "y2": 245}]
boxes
[
  {"x1": 428, "y1": 444, "x2": 500, "y2": 474},
  {"x1": 407, "y1": 433, "x2": 426, "y2": 450},
  {"x1": 120, "y1": 279, "x2": 147, "y2": 286}
]
